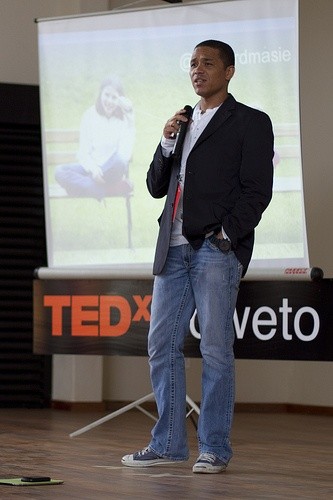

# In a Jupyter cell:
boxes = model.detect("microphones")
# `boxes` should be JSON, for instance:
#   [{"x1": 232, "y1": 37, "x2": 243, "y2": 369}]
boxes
[{"x1": 171, "y1": 105, "x2": 193, "y2": 157}]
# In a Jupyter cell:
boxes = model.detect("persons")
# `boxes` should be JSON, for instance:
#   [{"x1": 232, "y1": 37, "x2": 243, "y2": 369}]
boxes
[
  {"x1": 48, "y1": 78, "x2": 138, "y2": 197},
  {"x1": 120, "y1": 38, "x2": 274, "y2": 476}
]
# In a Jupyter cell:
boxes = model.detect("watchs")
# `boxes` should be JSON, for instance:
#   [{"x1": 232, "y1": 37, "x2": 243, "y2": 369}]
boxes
[{"x1": 209, "y1": 235, "x2": 232, "y2": 252}]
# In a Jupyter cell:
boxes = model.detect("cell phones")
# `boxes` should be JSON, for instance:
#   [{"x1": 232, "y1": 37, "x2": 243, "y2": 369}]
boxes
[{"x1": 21, "y1": 476, "x2": 51, "y2": 482}]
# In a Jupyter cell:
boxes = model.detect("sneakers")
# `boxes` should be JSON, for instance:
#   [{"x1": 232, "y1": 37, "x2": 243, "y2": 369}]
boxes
[
  {"x1": 193, "y1": 452, "x2": 227, "y2": 473},
  {"x1": 120, "y1": 446, "x2": 185, "y2": 466}
]
